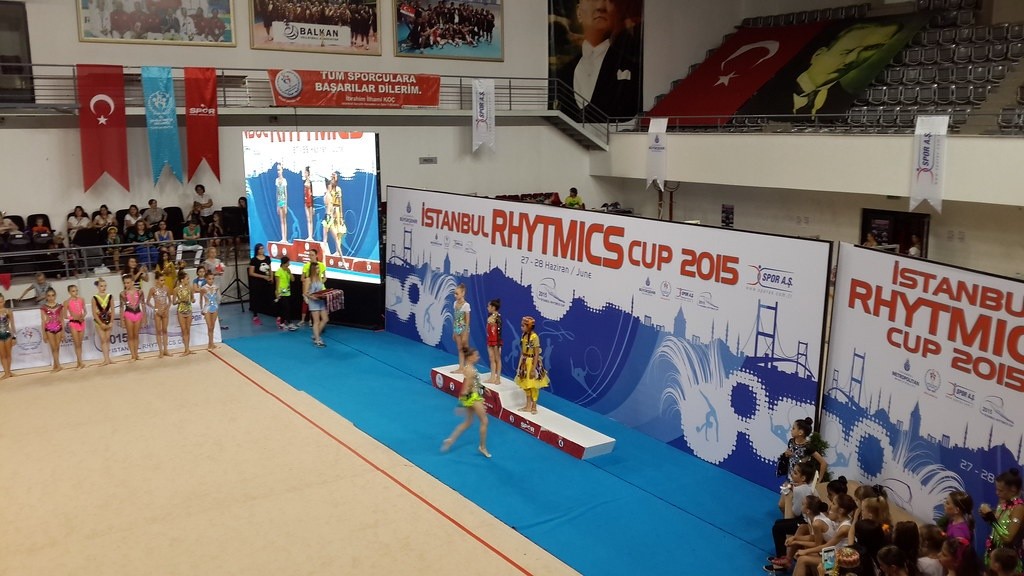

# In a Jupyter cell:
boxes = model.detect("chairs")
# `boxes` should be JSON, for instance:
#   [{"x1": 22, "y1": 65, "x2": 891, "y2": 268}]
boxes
[
  {"x1": 633, "y1": 0, "x2": 1024, "y2": 137},
  {"x1": 479, "y1": 192, "x2": 638, "y2": 216},
  {"x1": 0, "y1": 207, "x2": 250, "y2": 281}
]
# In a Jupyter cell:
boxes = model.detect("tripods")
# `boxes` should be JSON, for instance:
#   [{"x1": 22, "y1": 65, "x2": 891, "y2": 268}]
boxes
[{"x1": 221, "y1": 236, "x2": 249, "y2": 312}]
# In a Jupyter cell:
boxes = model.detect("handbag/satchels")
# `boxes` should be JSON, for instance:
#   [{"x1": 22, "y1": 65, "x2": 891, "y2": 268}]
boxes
[{"x1": 776, "y1": 452, "x2": 789, "y2": 476}]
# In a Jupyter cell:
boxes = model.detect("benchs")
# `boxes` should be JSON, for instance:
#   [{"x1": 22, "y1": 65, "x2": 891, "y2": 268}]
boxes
[{"x1": 781, "y1": 479, "x2": 1008, "y2": 576}]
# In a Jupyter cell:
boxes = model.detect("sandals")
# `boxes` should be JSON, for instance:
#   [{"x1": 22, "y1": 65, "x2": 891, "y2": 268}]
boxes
[{"x1": 772, "y1": 556, "x2": 793, "y2": 569}]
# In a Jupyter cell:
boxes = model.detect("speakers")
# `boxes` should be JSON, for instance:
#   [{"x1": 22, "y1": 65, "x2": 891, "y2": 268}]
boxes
[{"x1": 222, "y1": 207, "x2": 244, "y2": 236}]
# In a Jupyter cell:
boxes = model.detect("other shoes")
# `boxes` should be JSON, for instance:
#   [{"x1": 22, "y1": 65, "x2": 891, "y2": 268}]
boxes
[
  {"x1": 441, "y1": 439, "x2": 448, "y2": 452},
  {"x1": 517, "y1": 407, "x2": 531, "y2": 412},
  {"x1": 219, "y1": 317, "x2": 223, "y2": 320},
  {"x1": 767, "y1": 556, "x2": 775, "y2": 562},
  {"x1": 288, "y1": 323, "x2": 300, "y2": 330},
  {"x1": 253, "y1": 317, "x2": 263, "y2": 325},
  {"x1": 764, "y1": 565, "x2": 775, "y2": 572},
  {"x1": 309, "y1": 320, "x2": 313, "y2": 326},
  {"x1": 479, "y1": 446, "x2": 492, "y2": 457},
  {"x1": 531, "y1": 408, "x2": 537, "y2": 413},
  {"x1": 277, "y1": 319, "x2": 286, "y2": 324},
  {"x1": 279, "y1": 323, "x2": 289, "y2": 329},
  {"x1": 295, "y1": 320, "x2": 306, "y2": 326}
]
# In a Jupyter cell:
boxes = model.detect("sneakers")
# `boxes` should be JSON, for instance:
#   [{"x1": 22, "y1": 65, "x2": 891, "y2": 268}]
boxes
[
  {"x1": 314, "y1": 341, "x2": 327, "y2": 347},
  {"x1": 312, "y1": 336, "x2": 322, "y2": 339}
]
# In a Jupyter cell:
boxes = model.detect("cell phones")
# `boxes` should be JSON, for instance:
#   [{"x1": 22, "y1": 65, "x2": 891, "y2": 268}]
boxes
[{"x1": 822, "y1": 546, "x2": 836, "y2": 574}]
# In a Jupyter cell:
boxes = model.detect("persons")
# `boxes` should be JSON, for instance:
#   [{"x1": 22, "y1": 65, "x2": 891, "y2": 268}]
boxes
[
  {"x1": 40, "y1": 286, "x2": 67, "y2": 374},
  {"x1": 547, "y1": 0, "x2": 642, "y2": 122},
  {"x1": 262, "y1": 0, "x2": 378, "y2": 50},
  {"x1": 248, "y1": 243, "x2": 288, "y2": 326},
  {"x1": 0, "y1": 294, "x2": 18, "y2": 380},
  {"x1": 763, "y1": 415, "x2": 1023, "y2": 576},
  {"x1": 303, "y1": 166, "x2": 316, "y2": 241},
  {"x1": 0, "y1": 212, "x2": 24, "y2": 234},
  {"x1": 91, "y1": 279, "x2": 117, "y2": 367},
  {"x1": 173, "y1": 270, "x2": 197, "y2": 357},
  {"x1": 3, "y1": 271, "x2": 53, "y2": 308},
  {"x1": 734, "y1": 17, "x2": 907, "y2": 125},
  {"x1": 297, "y1": 250, "x2": 330, "y2": 347},
  {"x1": 452, "y1": 283, "x2": 471, "y2": 374},
  {"x1": 322, "y1": 173, "x2": 350, "y2": 270},
  {"x1": 69, "y1": 183, "x2": 226, "y2": 270},
  {"x1": 199, "y1": 270, "x2": 223, "y2": 349},
  {"x1": 238, "y1": 197, "x2": 249, "y2": 225},
  {"x1": 273, "y1": 256, "x2": 299, "y2": 331},
  {"x1": 543, "y1": 188, "x2": 584, "y2": 210},
  {"x1": 146, "y1": 271, "x2": 173, "y2": 358},
  {"x1": 119, "y1": 274, "x2": 148, "y2": 363},
  {"x1": 482, "y1": 298, "x2": 504, "y2": 385},
  {"x1": 397, "y1": 0, "x2": 495, "y2": 55},
  {"x1": 31, "y1": 217, "x2": 50, "y2": 235},
  {"x1": 907, "y1": 234, "x2": 923, "y2": 258},
  {"x1": 89, "y1": 1, "x2": 227, "y2": 45},
  {"x1": 275, "y1": 164, "x2": 290, "y2": 244},
  {"x1": 63, "y1": 285, "x2": 87, "y2": 371},
  {"x1": 121, "y1": 247, "x2": 223, "y2": 321},
  {"x1": 438, "y1": 346, "x2": 492, "y2": 457},
  {"x1": 514, "y1": 315, "x2": 550, "y2": 414},
  {"x1": 862, "y1": 231, "x2": 879, "y2": 248}
]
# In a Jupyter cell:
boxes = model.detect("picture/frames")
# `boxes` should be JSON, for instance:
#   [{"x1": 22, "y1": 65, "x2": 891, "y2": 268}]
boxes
[
  {"x1": 248, "y1": 0, "x2": 383, "y2": 57},
  {"x1": 75, "y1": 0, "x2": 237, "y2": 47},
  {"x1": 392, "y1": 0, "x2": 504, "y2": 62}
]
